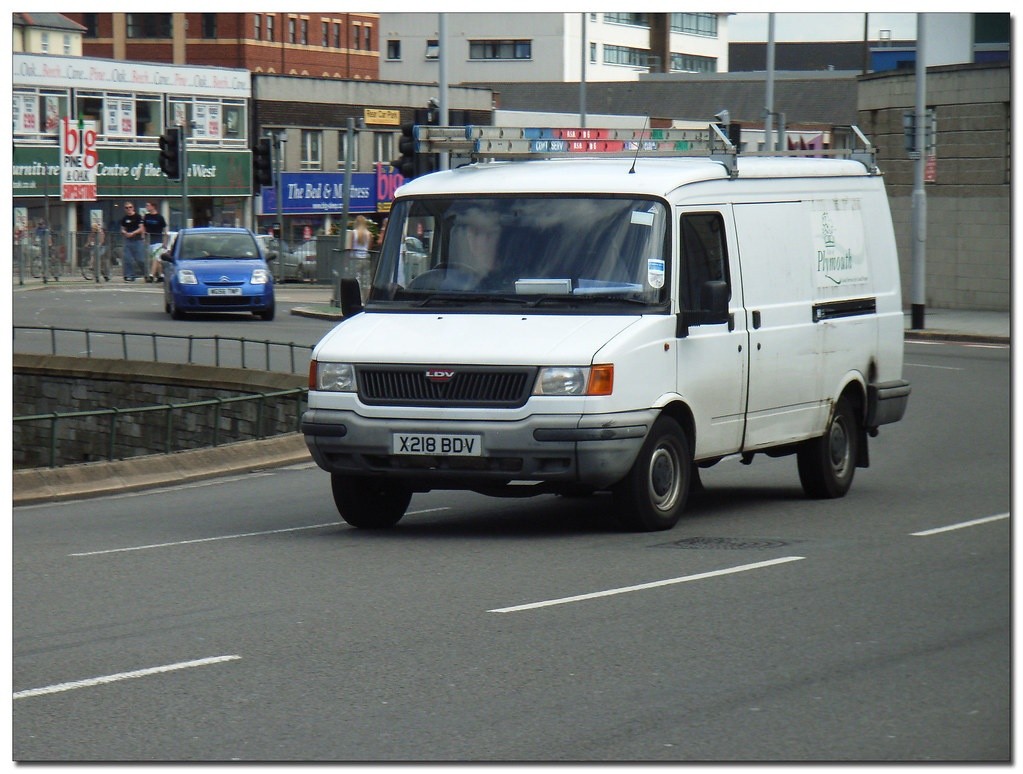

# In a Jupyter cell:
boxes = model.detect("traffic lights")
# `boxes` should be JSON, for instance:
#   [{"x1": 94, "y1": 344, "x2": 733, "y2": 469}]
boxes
[
  {"x1": 396, "y1": 122, "x2": 433, "y2": 180},
  {"x1": 252, "y1": 137, "x2": 274, "y2": 188},
  {"x1": 159, "y1": 127, "x2": 180, "y2": 182}
]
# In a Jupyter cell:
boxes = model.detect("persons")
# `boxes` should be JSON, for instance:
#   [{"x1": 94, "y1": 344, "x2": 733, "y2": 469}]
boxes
[
  {"x1": 377, "y1": 217, "x2": 389, "y2": 246},
  {"x1": 463, "y1": 214, "x2": 516, "y2": 290},
  {"x1": 182, "y1": 236, "x2": 212, "y2": 258},
  {"x1": 34, "y1": 220, "x2": 57, "y2": 262},
  {"x1": 141, "y1": 200, "x2": 167, "y2": 282},
  {"x1": 120, "y1": 202, "x2": 153, "y2": 282},
  {"x1": 86, "y1": 223, "x2": 105, "y2": 269},
  {"x1": 351, "y1": 216, "x2": 372, "y2": 302}
]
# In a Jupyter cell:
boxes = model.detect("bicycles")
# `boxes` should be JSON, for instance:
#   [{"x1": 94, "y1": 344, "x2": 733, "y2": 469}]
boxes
[
  {"x1": 31, "y1": 241, "x2": 64, "y2": 279},
  {"x1": 80, "y1": 242, "x2": 113, "y2": 282}
]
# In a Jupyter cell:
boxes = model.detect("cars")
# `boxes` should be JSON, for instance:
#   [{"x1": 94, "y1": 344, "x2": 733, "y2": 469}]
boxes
[
  {"x1": 160, "y1": 226, "x2": 278, "y2": 321},
  {"x1": 292, "y1": 235, "x2": 321, "y2": 283},
  {"x1": 257, "y1": 234, "x2": 305, "y2": 285},
  {"x1": 400, "y1": 235, "x2": 430, "y2": 283}
]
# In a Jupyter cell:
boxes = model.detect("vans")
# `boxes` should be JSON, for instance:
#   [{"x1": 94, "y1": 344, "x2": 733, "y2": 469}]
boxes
[{"x1": 301, "y1": 112, "x2": 913, "y2": 532}]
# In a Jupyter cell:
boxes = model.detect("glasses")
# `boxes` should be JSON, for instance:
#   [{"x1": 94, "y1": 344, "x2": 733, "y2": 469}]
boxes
[{"x1": 125, "y1": 206, "x2": 133, "y2": 209}]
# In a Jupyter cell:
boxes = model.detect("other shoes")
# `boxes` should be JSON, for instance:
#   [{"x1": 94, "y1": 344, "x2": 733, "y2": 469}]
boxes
[
  {"x1": 145, "y1": 276, "x2": 154, "y2": 282},
  {"x1": 157, "y1": 277, "x2": 165, "y2": 282}
]
